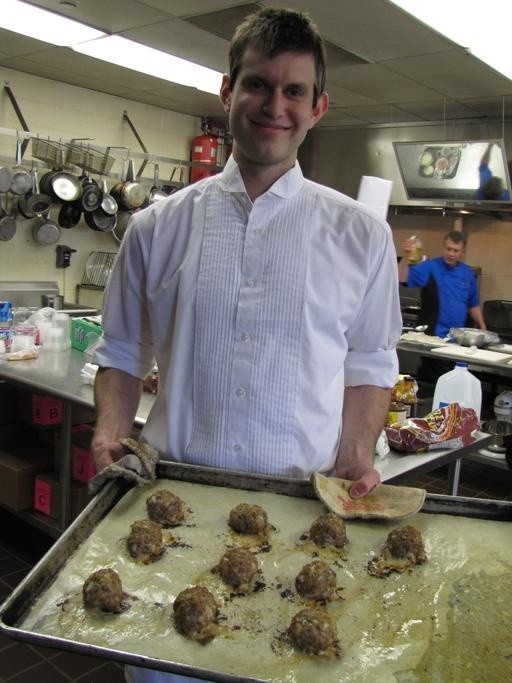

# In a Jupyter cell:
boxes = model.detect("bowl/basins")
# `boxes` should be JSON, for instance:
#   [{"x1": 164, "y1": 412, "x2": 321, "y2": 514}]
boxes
[
  {"x1": 454, "y1": 328, "x2": 500, "y2": 346},
  {"x1": 481, "y1": 420, "x2": 512, "y2": 453}
]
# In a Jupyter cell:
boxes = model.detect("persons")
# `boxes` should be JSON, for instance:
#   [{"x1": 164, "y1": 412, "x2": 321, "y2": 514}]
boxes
[
  {"x1": 87, "y1": 6, "x2": 404, "y2": 682},
  {"x1": 476, "y1": 142, "x2": 509, "y2": 201},
  {"x1": 397, "y1": 230, "x2": 488, "y2": 382}
]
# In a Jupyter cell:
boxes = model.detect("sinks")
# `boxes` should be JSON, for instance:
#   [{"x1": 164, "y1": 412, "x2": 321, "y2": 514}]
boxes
[{"x1": 11, "y1": 302, "x2": 97, "y2": 316}]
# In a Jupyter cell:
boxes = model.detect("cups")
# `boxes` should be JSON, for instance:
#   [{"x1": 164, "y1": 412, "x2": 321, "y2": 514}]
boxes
[{"x1": 44, "y1": 313, "x2": 73, "y2": 351}]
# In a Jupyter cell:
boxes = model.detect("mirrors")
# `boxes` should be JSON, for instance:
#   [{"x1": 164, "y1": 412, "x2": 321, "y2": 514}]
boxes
[{"x1": 391, "y1": 140, "x2": 512, "y2": 201}]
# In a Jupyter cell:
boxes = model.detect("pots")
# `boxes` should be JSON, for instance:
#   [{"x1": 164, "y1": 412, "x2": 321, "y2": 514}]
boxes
[{"x1": 1, "y1": 140, "x2": 184, "y2": 246}]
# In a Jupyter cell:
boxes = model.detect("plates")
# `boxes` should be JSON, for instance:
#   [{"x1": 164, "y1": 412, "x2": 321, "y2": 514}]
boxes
[{"x1": 434, "y1": 147, "x2": 451, "y2": 173}]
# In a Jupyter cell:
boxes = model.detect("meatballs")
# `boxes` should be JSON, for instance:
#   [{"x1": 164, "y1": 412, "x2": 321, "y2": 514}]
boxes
[
  {"x1": 127, "y1": 520, "x2": 163, "y2": 563},
  {"x1": 294, "y1": 561, "x2": 337, "y2": 606},
  {"x1": 309, "y1": 512, "x2": 347, "y2": 549},
  {"x1": 286, "y1": 608, "x2": 339, "y2": 654},
  {"x1": 387, "y1": 525, "x2": 424, "y2": 563},
  {"x1": 172, "y1": 585, "x2": 221, "y2": 642},
  {"x1": 229, "y1": 503, "x2": 268, "y2": 535},
  {"x1": 147, "y1": 489, "x2": 183, "y2": 527},
  {"x1": 211, "y1": 546, "x2": 261, "y2": 594},
  {"x1": 82, "y1": 568, "x2": 122, "y2": 612}
]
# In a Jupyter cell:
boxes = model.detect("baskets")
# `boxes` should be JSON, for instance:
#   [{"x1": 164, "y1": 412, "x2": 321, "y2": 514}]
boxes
[
  {"x1": 32, "y1": 136, "x2": 73, "y2": 165},
  {"x1": 64, "y1": 145, "x2": 117, "y2": 175}
]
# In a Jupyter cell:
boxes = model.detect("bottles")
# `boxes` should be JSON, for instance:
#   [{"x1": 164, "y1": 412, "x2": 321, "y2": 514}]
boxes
[{"x1": 400, "y1": 377, "x2": 418, "y2": 418}]
